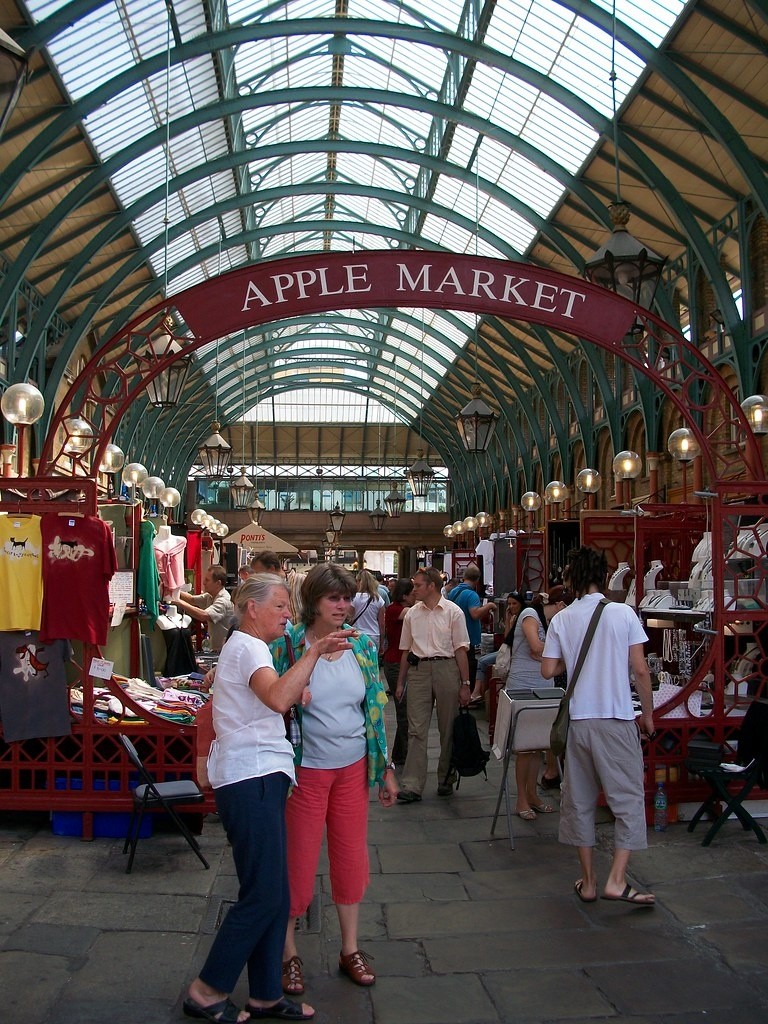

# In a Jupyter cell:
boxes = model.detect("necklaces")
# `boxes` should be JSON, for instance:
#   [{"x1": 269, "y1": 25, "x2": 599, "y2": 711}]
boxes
[
  {"x1": 312, "y1": 633, "x2": 334, "y2": 661},
  {"x1": 628, "y1": 586, "x2": 636, "y2": 596},
  {"x1": 694, "y1": 596, "x2": 714, "y2": 608},
  {"x1": 164, "y1": 613, "x2": 184, "y2": 635},
  {"x1": 611, "y1": 565, "x2": 629, "y2": 580},
  {"x1": 639, "y1": 593, "x2": 668, "y2": 607},
  {"x1": 661, "y1": 627, "x2": 687, "y2": 673},
  {"x1": 738, "y1": 529, "x2": 767, "y2": 548},
  {"x1": 689, "y1": 535, "x2": 712, "y2": 580},
  {"x1": 645, "y1": 564, "x2": 659, "y2": 578}
]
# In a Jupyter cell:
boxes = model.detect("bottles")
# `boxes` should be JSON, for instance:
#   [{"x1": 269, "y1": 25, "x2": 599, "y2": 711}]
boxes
[{"x1": 654, "y1": 783, "x2": 667, "y2": 830}]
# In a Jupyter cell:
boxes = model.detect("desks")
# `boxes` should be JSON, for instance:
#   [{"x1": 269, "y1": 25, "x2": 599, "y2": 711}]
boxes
[{"x1": 491, "y1": 687, "x2": 569, "y2": 850}]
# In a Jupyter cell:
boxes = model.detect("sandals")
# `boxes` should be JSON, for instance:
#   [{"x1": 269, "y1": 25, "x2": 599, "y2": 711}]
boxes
[
  {"x1": 281, "y1": 955, "x2": 305, "y2": 994},
  {"x1": 470, "y1": 696, "x2": 485, "y2": 704},
  {"x1": 339, "y1": 949, "x2": 376, "y2": 985}
]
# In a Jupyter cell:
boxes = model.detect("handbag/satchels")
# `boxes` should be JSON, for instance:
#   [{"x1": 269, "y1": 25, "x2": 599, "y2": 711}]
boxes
[
  {"x1": 496, "y1": 643, "x2": 510, "y2": 680},
  {"x1": 550, "y1": 698, "x2": 569, "y2": 756},
  {"x1": 284, "y1": 704, "x2": 302, "y2": 748}
]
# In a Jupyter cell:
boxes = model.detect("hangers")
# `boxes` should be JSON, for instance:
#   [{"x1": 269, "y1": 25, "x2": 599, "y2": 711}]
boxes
[
  {"x1": 43, "y1": 492, "x2": 104, "y2": 526},
  {"x1": 1, "y1": 495, "x2": 43, "y2": 522}
]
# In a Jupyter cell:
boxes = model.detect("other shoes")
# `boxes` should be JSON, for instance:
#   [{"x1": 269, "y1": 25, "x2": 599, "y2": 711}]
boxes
[
  {"x1": 386, "y1": 687, "x2": 391, "y2": 695},
  {"x1": 540, "y1": 775, "x2": 560, "y2": 789}
]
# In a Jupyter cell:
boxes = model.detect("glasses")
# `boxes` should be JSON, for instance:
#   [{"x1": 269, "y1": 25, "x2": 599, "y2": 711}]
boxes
[
  {"x1": 321, "y1": 594, "x2": 353, "y2": 602},
  {"x1": 418, "y1": 568, "x2": 436, "y2": 584}
]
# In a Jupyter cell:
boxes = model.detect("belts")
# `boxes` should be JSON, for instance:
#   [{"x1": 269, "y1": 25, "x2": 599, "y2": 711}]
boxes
[{"x1": 420, "y1": 655, "x2": 455, "y2": 661}]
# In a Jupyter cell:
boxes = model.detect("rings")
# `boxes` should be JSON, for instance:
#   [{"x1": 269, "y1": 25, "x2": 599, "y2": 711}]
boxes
[{"x1": 307, "y1": 691, "x2": 310, "y2": 693}]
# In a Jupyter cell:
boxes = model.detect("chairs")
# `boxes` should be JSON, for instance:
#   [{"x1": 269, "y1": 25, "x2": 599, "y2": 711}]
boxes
[
  {"x1": 117, "y1": 734, "x2": 211, "y2": 875},
  {"x1": 682, "y1": 698, "x2": 768, "y2": 847}
]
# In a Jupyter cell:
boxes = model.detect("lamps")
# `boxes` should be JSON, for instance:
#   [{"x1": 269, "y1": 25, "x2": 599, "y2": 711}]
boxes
[
  {"x1": 443, "y1": 452, "x2": 643, "y2": 536},
  {"x1": 191, "y1": 508, "x2": 229, "y2": 537},
  {"x1": 454, "y1": 136, "x2": 497, "y2": 451},
  {"x1": 135, "y1": 3, "x2": 196, "y2": 406},
  {"x1": 405, "y1": 296, "x2": 436, "y2": 497},
  {"x1": 231, "y1": 326, "x2": 256, "y2": 510},
  {"x1": 55, "y1": 417, "x2": 181, "y2": 508},
  {"x1": 199, "y1": 224, "x2": 233, "y2": 478},
  {"x1": 739, "y1": 392, "x2": 768, "y2": 435},
  {"x1": 369, "y1": 403, "x2": 385, "y2": 533},
  {"x1": 324, "y1": 499, "x2": 346, "y2": 551},
  {"x1": 668, "y1": 428, "x2": 701, "y2": 461},
  {"x1": 581, "y1": 0, "x2": 664, "y2": 334},
  {"x1": 384, "y1": 356, "x2": 406, "y2": 516},
  {"x1": 1, "y1": 382, "x2": 45, "y2": 423},
  {"x1": 247, "y1": 490, "x2": 264, "y2": 529}
]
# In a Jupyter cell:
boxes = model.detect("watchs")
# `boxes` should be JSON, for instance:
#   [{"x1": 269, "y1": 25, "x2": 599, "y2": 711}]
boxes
[
  {"x1": 386, "y1": 762, "x2": 396, "y2": 769},
  {"x1": 462, "y1": 679, "x2": 470, "y2": 685}
]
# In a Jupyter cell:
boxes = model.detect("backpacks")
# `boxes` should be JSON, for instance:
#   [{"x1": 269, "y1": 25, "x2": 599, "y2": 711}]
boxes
[{"x1": 442, "y1": 704, "x2": 490, "y2": 790}]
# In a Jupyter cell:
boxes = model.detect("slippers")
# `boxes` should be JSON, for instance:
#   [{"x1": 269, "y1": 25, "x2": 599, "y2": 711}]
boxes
[
  {"x1": 600, "y1": 884, "x2": 655, "y2": 904},
  {"x1": 515, "y1": 807, "x2": 536, "y2": 820},
  {"x1": 245, "y1": 997, "x2": 313, "y2": 1020},
  {"x1": 531, "y1": 802, "x2": 553, "y2": 813},
  {"x1": 575, "y1": 878, "x2": 597, "y2": 903},
  {"x1": 183, "y1": 996, "x2": 249, "y2": 1024}
]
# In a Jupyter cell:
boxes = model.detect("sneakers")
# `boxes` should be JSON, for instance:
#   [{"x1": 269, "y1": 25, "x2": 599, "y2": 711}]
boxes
[
  {"x1": 397, "y1": 789, "x2": 422, "y2": 801},
  {"x1": 437, "y1": 783, "x2": 453, "y2": 795}
]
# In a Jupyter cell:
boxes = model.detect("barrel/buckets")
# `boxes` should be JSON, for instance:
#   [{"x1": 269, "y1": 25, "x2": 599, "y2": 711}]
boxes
[{"x1": 480, "y1": 633, "x2": 494, "y2": 655}]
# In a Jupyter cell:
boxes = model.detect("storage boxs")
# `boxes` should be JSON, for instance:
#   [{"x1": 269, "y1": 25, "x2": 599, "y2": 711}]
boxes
[{"x1": 48, "y1": 752, "x2": 156, "y2": 842}]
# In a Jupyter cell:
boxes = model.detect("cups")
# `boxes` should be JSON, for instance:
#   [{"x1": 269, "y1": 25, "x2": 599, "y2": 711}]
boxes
[{"x1": 526, "y1": 591, "x2": 533, "y2": 600}]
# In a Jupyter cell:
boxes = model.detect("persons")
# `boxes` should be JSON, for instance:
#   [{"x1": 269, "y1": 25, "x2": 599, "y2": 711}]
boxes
[
  {"x1": 397, "y1": 567, "x2": 473, "y2": 800},
  {"x1": 439, "y1": 564, "x2": 535, "y2": 704},
  {"x1": 542, "y1": 544, "x2": 657, "y2": 904},
  {"x1": 167, "y1": 549, "x2": 306, "y2": 653},
  {"x1": 503, "y1": 585, "x2": 572, "y2": 820},
  {"x1": 152, "y1": 525, "x2": 188, "y2": 589},
  {"x1": 560, "y1": 568, "x2": 572, "y2": 589},
  {"x1": 268, "y1": 560, "x2": 396, "y2": 996},
  {"x1": 345, "y1": 567, "x2": 423, "y2": 764},
  {"x1": 156, "y1": 604, "x2": 197, "y2": 676},
  {"x1": 172, "y1": 569, "x2": 358, "y2": 1024}
]
